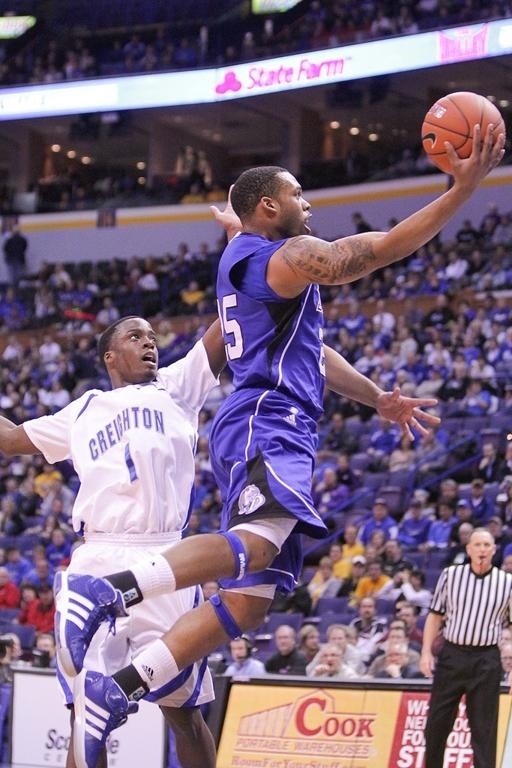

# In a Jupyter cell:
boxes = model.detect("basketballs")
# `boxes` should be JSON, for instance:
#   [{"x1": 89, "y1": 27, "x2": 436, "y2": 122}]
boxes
[{"x1": 422, "y1": 93, "x2": 504, "y2": 177}]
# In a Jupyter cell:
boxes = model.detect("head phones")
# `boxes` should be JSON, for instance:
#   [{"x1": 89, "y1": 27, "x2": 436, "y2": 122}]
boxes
[{"x1": 227, "y1": 635, "x2": 252, "y2": 655}]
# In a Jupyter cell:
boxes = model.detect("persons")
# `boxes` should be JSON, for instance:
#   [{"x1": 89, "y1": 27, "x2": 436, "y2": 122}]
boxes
[{"x1": 0, "y1": 0, "x2": 511, "y2": 768}]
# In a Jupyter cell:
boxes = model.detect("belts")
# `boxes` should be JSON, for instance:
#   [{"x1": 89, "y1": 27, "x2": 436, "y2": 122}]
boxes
[{"x1": 445, "y1": 642, "x2": 498, "y2": 651}]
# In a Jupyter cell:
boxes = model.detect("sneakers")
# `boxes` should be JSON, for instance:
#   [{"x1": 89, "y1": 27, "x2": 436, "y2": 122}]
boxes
[
  {"x1": 73, "y1": 668, "x2": 138, "y2": 768},
  {"x1": 52, "y1": 571, "x2": 129, "y2": 677}
]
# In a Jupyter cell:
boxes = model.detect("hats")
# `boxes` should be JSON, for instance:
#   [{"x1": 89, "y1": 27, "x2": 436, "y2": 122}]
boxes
[
  {"x1": 352, "y1": 556, "x2": 366, "y2": 564},
  {"x1": 374, "y1": 478, "x2": 502, "y2": 524}
]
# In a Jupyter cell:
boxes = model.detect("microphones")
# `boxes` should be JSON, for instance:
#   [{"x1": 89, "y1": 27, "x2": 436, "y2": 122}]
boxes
[{"x1": 237, "y1": 654, "x2": 250, "y2": 659}]
